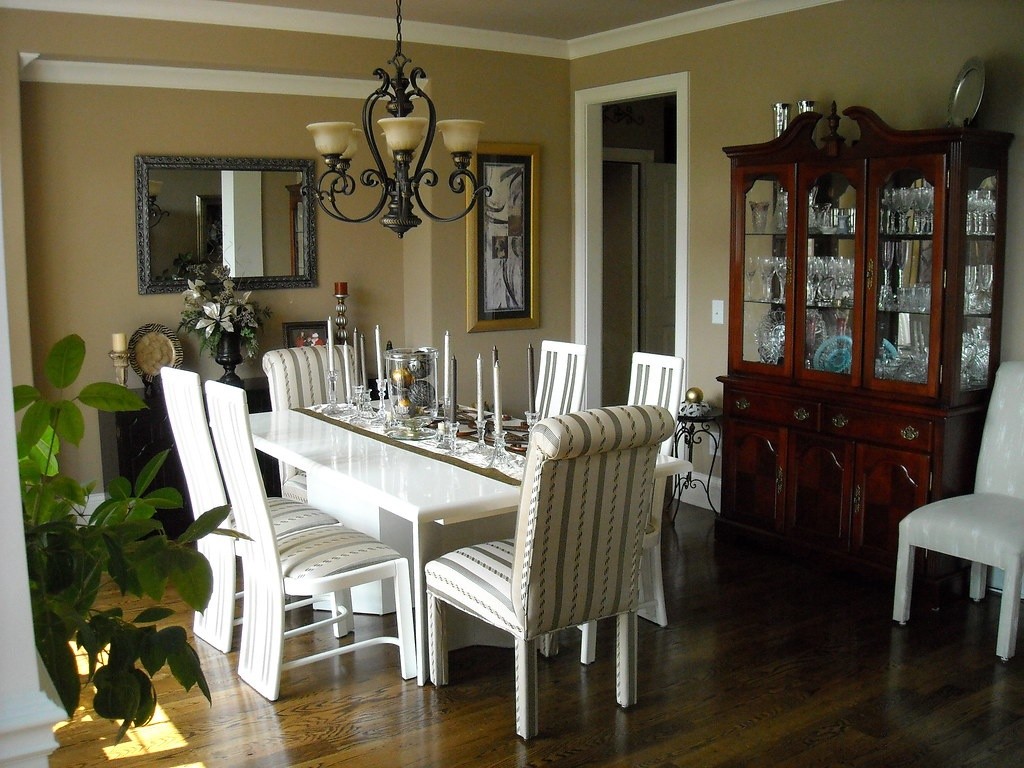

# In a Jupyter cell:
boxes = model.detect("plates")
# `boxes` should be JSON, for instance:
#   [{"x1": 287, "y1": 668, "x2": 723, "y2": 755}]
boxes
[
  {"x1": 947, "y1": 57, "x2": 986, "y2": 127},
  {"x1": 127, "y1": 322, "x2": 183, "y2": 382}
]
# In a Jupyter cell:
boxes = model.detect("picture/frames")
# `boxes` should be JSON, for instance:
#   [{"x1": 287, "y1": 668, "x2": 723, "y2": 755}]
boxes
[
  {"x1": 464, "y1": 140, "x2": 541, "y2": 334},
  {"x1": 282, "y1": 320, "x2": 329, "y2": 348},
  {"x1": 195, "y1": 193, "x2": 222, "y2": 265}
]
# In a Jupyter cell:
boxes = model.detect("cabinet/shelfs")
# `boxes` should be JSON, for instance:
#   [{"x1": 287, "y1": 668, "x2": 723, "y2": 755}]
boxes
[
  {"x1": 97, "y1": 376, "x2": 388, "y2": 550},
  {"x1": 284, "y1": 180, "x2": 307, "y2": 274},
  {"x1": 708, "y1": 99, "x2": 1018, "y2": 580}
]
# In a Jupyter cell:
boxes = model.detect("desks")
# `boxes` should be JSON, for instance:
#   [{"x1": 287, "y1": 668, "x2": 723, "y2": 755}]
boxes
[
  {"x1": 247, "y1": 394, "x2": 694, "y2": 685},
  {"x1": 665, "y1": 403, "x2": 722, "y2": 522}
]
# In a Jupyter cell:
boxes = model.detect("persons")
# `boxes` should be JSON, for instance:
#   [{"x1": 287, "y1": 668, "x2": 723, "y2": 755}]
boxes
[{"x1": 296, "y1": 331, "x2": 322, "y2": 347}]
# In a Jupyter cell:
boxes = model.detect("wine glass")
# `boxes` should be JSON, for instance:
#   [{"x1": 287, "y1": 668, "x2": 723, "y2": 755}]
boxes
[{"x1": 746, "y1": 187, "x2": 996, "y2": 390}]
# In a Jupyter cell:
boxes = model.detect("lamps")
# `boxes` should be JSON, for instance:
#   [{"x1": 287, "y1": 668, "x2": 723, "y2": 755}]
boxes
[
  {"x1": 147, "y1": 179, "x2": 170, "y2": 230},
  {"x1": 301, "y1": 0, "x2": 494, "y2": 241}
]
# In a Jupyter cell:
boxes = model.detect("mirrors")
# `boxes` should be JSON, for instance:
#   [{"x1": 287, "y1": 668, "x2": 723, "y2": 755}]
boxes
[
  {"x1": 944, "y1": 55, "x2": 987, "y2": 127},
  {"x1": 132, "y1": 154, "x2": 319, "y2": 296}
]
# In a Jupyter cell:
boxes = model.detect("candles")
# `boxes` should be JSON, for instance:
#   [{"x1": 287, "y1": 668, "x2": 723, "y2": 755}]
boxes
[
  {"x1": 335, "y1": 281, "x2": 347, "y2": 295},
  {"x1": 386, "y1": 340, "x2": 392, "y2": 351},
  {"x1": 493, "y1": 360, "x2": 504, "y2": 434},
  {"x1": 327, "y1": 315, "x2": 337, "y2": 372},
  {"x1": 476, "y1": 352, "x2": 485, "y2": 422},
  {"x1": 444, "y1": 330, "x2": 450, "y2": 400},
  {"x1": 111, "y1": 333, "x2": 129, "y2": 352},
  {"x1": 491, "y1": 345, "x2": 499, "y2": 366},
  {"x1": 375, "y1": 325, "x2": 385, "y2": 380},
  {"x1": 526, "y1": 342, "x2": 536, "y2": 413},
  {"x1": 451, "y1": 354, "x2": 458, "y2": 423},
  {"x1": 352, "y1": 326, "x2": 359, "y2": 385},
  {"x1": 359, "y1": 333, "x2": 369, "y2": 390},
  {"x1": 344, "y1": 340, "x2": 353, "y2": 397}
]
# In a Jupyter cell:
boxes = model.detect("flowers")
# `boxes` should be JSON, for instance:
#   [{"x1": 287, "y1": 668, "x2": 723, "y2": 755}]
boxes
[
  {"x1": 174, "y1": 264, "x2": 273, "y2": 367},
  {"x1": 155, "y1": 250, "x2": 232, "y2": 281}
]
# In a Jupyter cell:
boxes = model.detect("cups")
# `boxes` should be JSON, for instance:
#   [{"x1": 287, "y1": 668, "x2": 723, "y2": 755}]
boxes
[
  {"x1": 797, "y1": 100, "x2": 815, "y2": 114},
  {"x1": 773, "y1": 102, "x2": 790, "y2": 140},
  {"x1": 383, "y1": 348, "x2": 440, "y2": 440},
  {"x1": 749, "y1": 201, "x2": 769, "y2": 233}
]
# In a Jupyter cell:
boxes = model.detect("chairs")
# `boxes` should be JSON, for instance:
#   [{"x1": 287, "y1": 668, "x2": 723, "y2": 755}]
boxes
[
  {"x1": 423, "y1": 402, "x2": 675, "y2": 742},
  {"x1": 261, "y1": 342, "x2": 367, "y2": 503},
  {"x1": 571, "y1": 351, "x2": 686, "y2": 667},
  {"x1": 535, "y1": 339, "x2": 590, "y2": 422},
  {"x1": 158, "y1": 366, "x2": 355, "y2": 659},
  {"x1": 890, "y1": 359, "x2": 1024, "y2": 663},
  {"x1": 205, "y1": 380, "x2": 417, "y2": 700}
]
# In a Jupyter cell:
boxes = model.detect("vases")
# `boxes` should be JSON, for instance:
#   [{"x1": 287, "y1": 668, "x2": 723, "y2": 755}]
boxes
[{"x1": 214, "y1": 323, "x2": 244, "y2": 387}]
